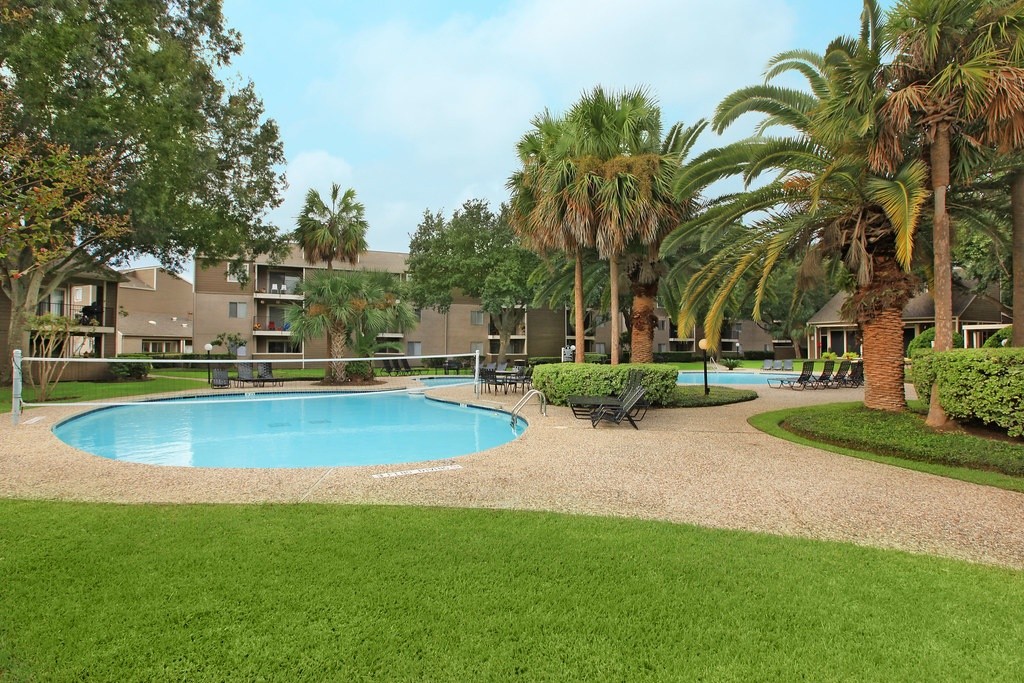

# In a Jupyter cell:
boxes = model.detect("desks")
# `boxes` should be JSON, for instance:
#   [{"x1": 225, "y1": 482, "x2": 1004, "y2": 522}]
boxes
[{"x1": 496, "y1": 370, "x2": 517, "y2": 393}]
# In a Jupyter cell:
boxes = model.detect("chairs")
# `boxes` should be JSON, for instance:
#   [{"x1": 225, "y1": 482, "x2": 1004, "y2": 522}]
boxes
[
  {"x1": 379, "y1": 358, "x2": 474, "y2": 376},
  {"x1": 478, "y1": 361, "x2": 533, "y2": 395},
  {"x1": 568, "y1": 368, "x2": 649, "y2": 429},
  {"x1": 211, "y1": 361, "x2": 284, "y2": 389},
  {"x1": 270, "y1": 283, "x2": 278, "y2": 293},
  {"x1": 764, "y1": 359, "x2": 863, "y2": 391},
  {"x1": 279, "y1": 284, "x2": 286, "y2": 293},
  {"x1": 268, "y1": 321, "x2": 274, "y2": 330}
]
